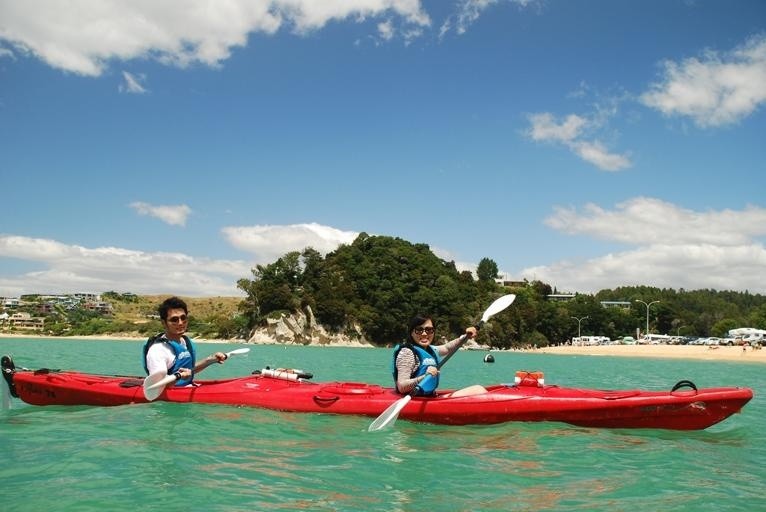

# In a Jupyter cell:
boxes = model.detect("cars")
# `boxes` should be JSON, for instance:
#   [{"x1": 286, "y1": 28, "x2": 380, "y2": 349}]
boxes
[
  {"x1": 692, "y1": 334, "x2": 766, "y2": 345},
  {"x1": 572, "y1": 333, "x2": 691, "y2": 346}
]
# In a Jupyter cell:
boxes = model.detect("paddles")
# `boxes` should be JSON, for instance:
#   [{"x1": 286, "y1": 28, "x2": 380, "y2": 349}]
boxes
[
  {"x1": 369, "y1": 293, "x2": 516, "y2": 431},
  {"x1": 143, "y1": 348, "x2": 250, "y2": 401}
]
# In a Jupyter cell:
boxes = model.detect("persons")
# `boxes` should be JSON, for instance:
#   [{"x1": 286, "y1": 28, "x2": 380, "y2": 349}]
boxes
[
  {"x1": 391, "y1": 314, "x2": 488, "y2": 399},
  {"x1": 141, "y1": 297, "x2": 225, "y2": 388},
  {"x1": 751, "y1": 340, "x2": 756, "y2": 352},
  {"x1": 755, "y1": 342, "x2": 762, "y2": 350},
  {"x1": 741, "y1": 342, "x2": 749, "y2": 354}
]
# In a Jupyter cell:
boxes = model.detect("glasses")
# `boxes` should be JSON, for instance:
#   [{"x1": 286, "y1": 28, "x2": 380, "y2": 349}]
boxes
[
  {"x1": 166, "y1": 314, "x2": 185, "y2": 322},
  {"x1": 414, "y1": 327, "x2": 434, "y2": 334}
]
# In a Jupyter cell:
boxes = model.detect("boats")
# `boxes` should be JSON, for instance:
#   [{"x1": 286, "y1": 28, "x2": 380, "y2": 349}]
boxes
[{"x1": 0, "y1": 356, "x2": 744, "y2": 431}]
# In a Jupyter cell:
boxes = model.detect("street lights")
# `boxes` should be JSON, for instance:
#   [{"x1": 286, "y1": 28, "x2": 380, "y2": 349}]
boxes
[
  {"x1": 571, "y1": 316, "x2": 588, "y2": 337},
  {"x1": 636, "y1": 299, "x2": 660, "y2": 333}
]
